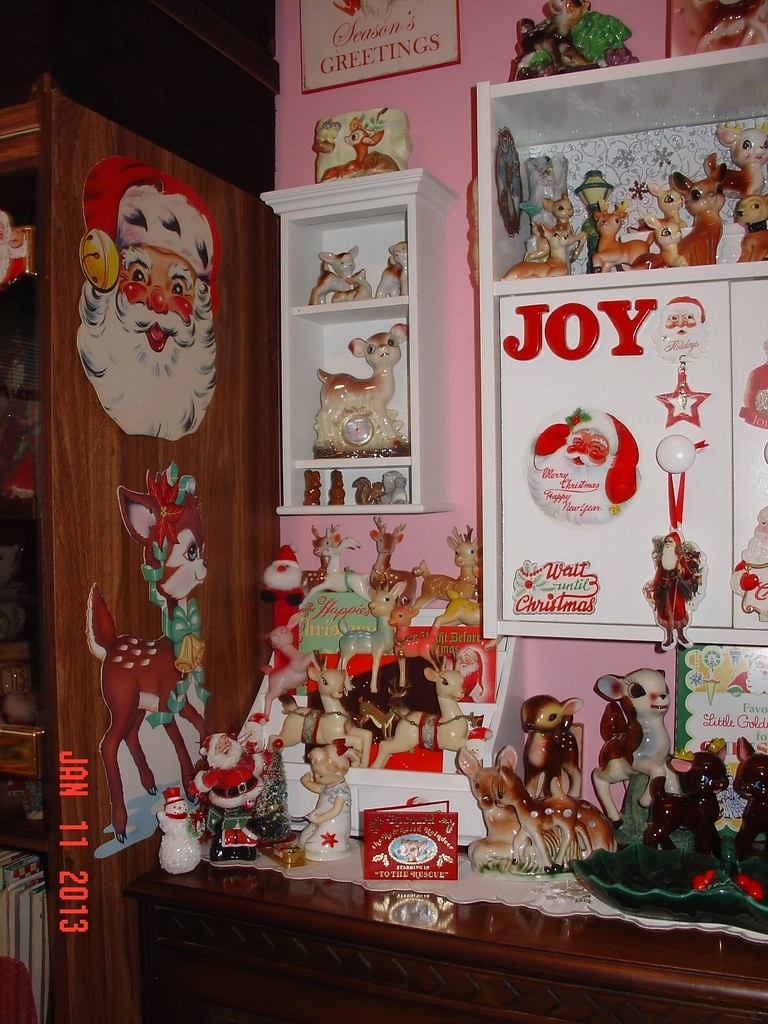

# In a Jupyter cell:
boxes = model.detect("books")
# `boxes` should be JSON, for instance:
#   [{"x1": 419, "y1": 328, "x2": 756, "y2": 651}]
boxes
[{"x1": 0, "y1": 848, "x2": 49, "y2": 1024}]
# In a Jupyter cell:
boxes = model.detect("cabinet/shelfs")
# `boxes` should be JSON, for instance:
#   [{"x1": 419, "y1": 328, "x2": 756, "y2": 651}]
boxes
[
  {"x1": 118, "y1": 831, "x2": 768, "y2": 1023},
  {"x1": 471, "y1": 40, "x2": 768, "y2": 646},
  {"x1": 258, "y1": 166, "x2": 458, "y2": 516},
  {"x1": 0, "y1": 70, "x2": 283, "y2": 1024}
]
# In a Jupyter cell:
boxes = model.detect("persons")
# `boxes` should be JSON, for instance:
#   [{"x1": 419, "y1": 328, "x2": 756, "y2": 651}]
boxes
[
  {"x1": 187, "y1": 731, "x2": 277, "y2": 816},
  {"x1": 296, "y1": 739, "x2": 362, "y2": 863}
]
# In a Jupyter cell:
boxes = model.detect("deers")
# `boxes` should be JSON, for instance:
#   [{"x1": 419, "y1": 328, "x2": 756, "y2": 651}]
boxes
[
  {"x1": 457, "y1": 668, "x2": 768, "y2": 874},
  {"x1": 309, "y1": 323, "x2": 408, "y2": 453},
  {"x1": 308, "y1": 242, "x2": 408, "y2": 305},
  {"x1": 500, "y1": 119, "x2": 767, "y2": 282},
  {"x1": 260, "y1": 516, "x2": 484, "y2": 770}
]
